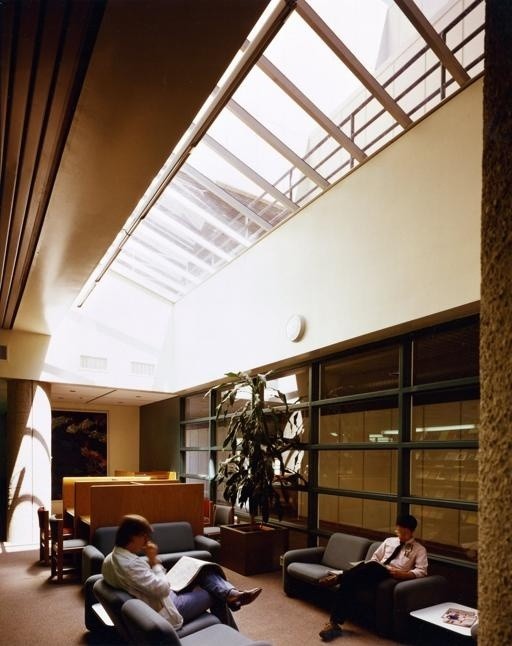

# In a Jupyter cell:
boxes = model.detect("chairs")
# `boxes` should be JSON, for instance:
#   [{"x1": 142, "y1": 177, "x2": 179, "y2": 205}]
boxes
[
  {"x1": 202, "y1": 500, "x2": 216, "y2": 527},
  {"x1": 37, "y1": 506, "x2": 73, "y2": 566},
  {"x1": 49, "y1": 512, "x2": 90, "y2": 584},
  {"x1": 203, "y1": 504, "x2": 234, "y2": 538}
]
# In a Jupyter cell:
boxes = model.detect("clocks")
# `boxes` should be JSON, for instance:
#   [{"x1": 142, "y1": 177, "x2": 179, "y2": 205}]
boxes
[{"x1": 285, "y1": 314, "x2": 305, "y2": 343}]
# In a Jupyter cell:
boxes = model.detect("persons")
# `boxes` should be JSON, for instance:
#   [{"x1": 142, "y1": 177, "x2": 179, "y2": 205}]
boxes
[
  {"x1": 319, "y1": 514, "x2": 428, "y2": 639},
  {"x1": 102, "y1": 514, "x2": 262, "y2": 632}
]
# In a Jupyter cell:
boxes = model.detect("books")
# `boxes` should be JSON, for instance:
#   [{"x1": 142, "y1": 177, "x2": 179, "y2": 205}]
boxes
[
  {"x1": 166, "y1": 555, "x2": 228, "y2": 594},
  {"x1": 349, "y1": 560, "x2": 391, "y2": 579}
]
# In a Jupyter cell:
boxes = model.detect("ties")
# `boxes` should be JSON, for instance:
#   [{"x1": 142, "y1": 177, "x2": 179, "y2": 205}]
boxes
[{"x1": 384, "y1": 542, "x2": 405, "y2": 565}]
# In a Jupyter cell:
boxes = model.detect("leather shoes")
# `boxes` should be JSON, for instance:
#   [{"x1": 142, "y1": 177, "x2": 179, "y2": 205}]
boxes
[
  {"x1": 319, "y1": 571, "x2": 337, "y2": 587},
  {"x1": 319, "y1": 623, "x2": 341, "y2": 640},
  {"x1": 225, "y1": 587, "x2": 262, "y2": 611}
]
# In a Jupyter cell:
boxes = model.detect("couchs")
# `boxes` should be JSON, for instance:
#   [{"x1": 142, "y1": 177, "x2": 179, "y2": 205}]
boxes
[
  {"x1": 84, "y1": 574, "x2": 274, "y2": 645},
  {"x1": 82, "y1": 521, "x2": 222, "y2": 594},
  {"x1": 282, "y1": 533, "x2": 446, "y2": 643}
]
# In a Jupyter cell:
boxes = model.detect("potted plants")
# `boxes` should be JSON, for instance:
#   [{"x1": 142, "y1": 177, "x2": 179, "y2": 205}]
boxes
[{"x1": 208, "y1": 368, "x2": 309, "y2": 577}]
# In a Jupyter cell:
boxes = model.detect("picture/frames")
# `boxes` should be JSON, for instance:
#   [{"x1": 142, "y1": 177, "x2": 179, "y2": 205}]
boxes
[{"x1": 51, "y1": 407, "x2": 111, "y2": 504}]
